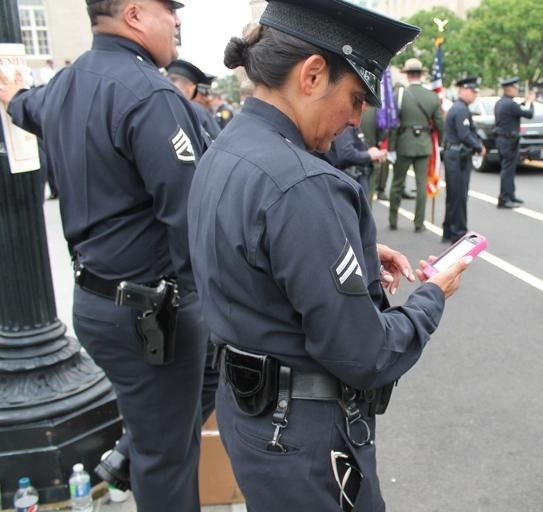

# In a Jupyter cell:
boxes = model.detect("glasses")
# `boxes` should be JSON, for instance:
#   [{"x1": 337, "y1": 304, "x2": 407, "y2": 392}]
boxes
[{"x1": 330, "y1": 450, "x2": 363, "y2": 509}]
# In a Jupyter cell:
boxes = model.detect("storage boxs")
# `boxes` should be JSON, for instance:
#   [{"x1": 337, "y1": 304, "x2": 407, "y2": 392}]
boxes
[{"x1": 196, "y1": 410, "x2": 247, "y2": 507}]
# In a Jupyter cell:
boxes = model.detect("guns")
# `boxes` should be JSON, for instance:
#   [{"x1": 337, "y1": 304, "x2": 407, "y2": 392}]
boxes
[{"x1": 115, "y1": 278, "x2": 182, "y2": 366}]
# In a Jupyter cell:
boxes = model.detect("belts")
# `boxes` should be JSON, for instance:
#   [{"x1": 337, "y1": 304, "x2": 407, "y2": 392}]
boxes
[
  {"x1": 285, "y1": 371, "x2": 342, "y2": 402},
  {"x1": 72, "y1": 267, "x2": 116, "y2": 299}
]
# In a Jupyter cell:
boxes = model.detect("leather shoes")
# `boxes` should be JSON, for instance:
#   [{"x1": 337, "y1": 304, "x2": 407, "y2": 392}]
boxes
[
  {"x1": 499, "y1": 200, "x2": 519, "y2": 208},
  {"x1": 94, "y1": 448, "x2": 131, "y2": 492},
  {"x1": 515, "y1": 199, "x2": 523, "y2": 203}
]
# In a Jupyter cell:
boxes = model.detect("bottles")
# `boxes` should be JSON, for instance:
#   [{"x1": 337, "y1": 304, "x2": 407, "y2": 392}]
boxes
[
  {"x1": 11, "y1": 476, "x2": 40, "y2": 512},
  {"x1": 68, "y1": 462, "x2": 96, "y2": 511}
]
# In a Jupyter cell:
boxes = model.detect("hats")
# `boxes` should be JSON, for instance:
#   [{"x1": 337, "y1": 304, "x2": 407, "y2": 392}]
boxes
[
  {"x1": 166, "y1": 60, "x2": 217, "y2": 96},
  {"x1": 500, "y1": 76, "x2": 519, "y2": 89},
  {"x1": 400, "y1": 58, "x2": 428, "y2": 73},
  {"x1": 454, "y1": 76, "x2": 482, "y2": 92},
  {"x1": 258, "y1": 2, "x2": 421, "y2": 108}
]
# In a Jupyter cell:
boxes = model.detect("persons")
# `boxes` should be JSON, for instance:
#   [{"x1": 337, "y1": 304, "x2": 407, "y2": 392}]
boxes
[
  {"x1": 186, "y1": 0, "x2": 474, "y2": 512},
  {"x1": 0, "y1": 0, "x2": 218, "y2": 512}
]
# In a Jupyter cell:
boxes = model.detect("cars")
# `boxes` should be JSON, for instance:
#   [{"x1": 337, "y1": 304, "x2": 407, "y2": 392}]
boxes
[{"x1": 469, "y1": 96, "x2": 542, "y2": 174}]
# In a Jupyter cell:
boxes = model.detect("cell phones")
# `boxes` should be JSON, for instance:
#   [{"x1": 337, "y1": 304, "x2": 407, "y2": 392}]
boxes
[{"x1": 422, "y1": 231, "x2": 488, "y2": 281}]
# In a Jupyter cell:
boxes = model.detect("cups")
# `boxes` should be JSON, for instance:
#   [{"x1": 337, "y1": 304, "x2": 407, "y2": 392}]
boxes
[{"x1": 102, "y1": 449, "x2": 132, "y2": 502}]
